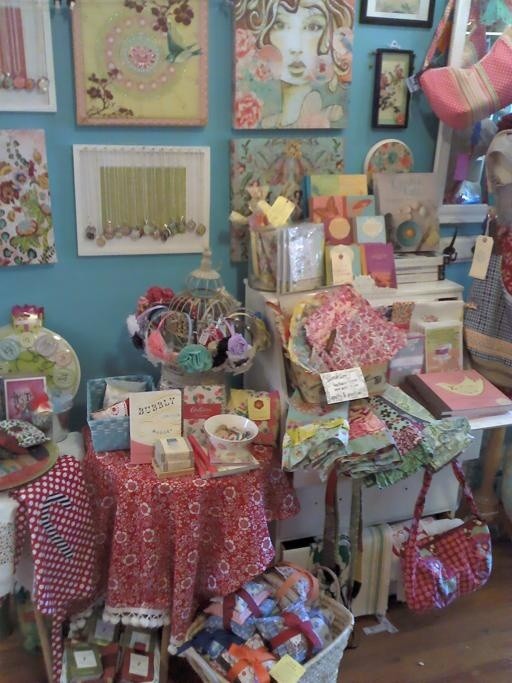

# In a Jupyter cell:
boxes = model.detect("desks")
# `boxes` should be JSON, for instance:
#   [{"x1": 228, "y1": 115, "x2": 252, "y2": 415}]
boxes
[
  {"x1": 85, "y1": 426, "x2": 297, "y2": 683},
  {"x1": 0, "y1": 431, "x2": 94, "y2": 683}
]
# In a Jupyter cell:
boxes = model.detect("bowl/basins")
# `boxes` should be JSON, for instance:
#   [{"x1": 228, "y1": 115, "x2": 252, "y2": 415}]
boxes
[{"x1": 203, "y1": 414, "x2": 260, "y2": 454}]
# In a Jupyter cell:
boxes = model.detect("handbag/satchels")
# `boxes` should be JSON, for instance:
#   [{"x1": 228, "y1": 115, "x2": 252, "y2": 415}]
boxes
[
  {"x1": 464, "y1": 216, "x2": 512, "y2": 375},
  {"x1": 419, "y1": 0, "x2": 512, "y2": 131},
  {"x1": 400, "y1": 458, "x2": 493, "y2": 613}
]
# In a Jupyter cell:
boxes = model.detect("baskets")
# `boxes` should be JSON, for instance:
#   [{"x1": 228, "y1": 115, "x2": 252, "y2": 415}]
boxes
[
  {"x1": 184, "y1": 566, "x2": 356, "y2": 683},
  {"x1": 86, "y1": 375, "x2": 156, "y2": 452}
]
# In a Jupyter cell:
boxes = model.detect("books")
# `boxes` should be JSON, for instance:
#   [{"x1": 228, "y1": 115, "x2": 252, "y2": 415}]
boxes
[
  {"x1": 275, "y1": 174, "x2": 447, "y2": 294},
  {"x1": 187, "y1": 434, "x2": 259, "y2": 480},
  {"x1": 399, "y1": 300, "x2": 512, "y2": 420}
]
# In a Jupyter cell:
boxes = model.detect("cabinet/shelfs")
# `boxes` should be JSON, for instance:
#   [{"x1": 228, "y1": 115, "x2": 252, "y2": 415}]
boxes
[{"x1": 242, "y1": 279, "x2": 464, "y2": 562}]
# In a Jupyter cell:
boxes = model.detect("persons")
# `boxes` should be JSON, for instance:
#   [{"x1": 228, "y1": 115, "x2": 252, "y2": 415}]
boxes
[{"x1": 232, "y1": 0, "x2": 355, "y2": 130}]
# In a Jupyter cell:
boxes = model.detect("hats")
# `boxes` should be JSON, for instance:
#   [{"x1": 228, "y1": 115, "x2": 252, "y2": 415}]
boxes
[
  {"x1": 471, "y1": 119, "x2": 498, "y2": 159},
  {"x1": 486, "y1": 129, "x2": 512, "y2": 228}
]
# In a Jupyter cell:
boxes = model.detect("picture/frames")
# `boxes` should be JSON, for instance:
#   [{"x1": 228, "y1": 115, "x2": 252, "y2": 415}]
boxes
[
  {"x1": 433, "y1": 0, "x2": 512, "y2": 225},
  {"x1": 72, "y1": 145, "x2": 211, "y2": 257},
  {"x1": 360, "y1": 0, "x2": 435, "y2": 28},
  {"x1": 373, "y1": 49, "x2": 413, "y2": 128},
  {"x1": 4, "y1": 377, "x2": 46, "y2": 423},
  {"x1": 69, "y1": 0, "x2": 208, "y2": 126},
  {"x1": 363, "y1": 139, "x2": 414, "y2": 193},
  {"x1": 0, "y1": 0, "x2": 58, "y2": 112}
]
